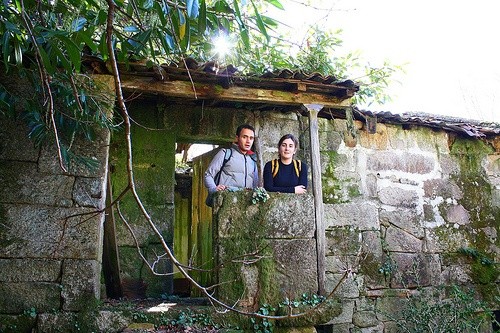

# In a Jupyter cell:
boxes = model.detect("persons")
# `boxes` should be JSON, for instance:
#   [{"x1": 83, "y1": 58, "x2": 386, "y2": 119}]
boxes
[
  {"x1": 264, "y1": 134, "x2": 308, "y2": 194},
  {"x1": 203, "y1": 124, "x2": 259, "y2": 193}
]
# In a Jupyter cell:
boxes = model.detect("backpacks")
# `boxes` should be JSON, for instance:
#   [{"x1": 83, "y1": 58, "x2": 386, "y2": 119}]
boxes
[{"x1": 202, "y1": 148, "x2": 232, "y2": 207}]
